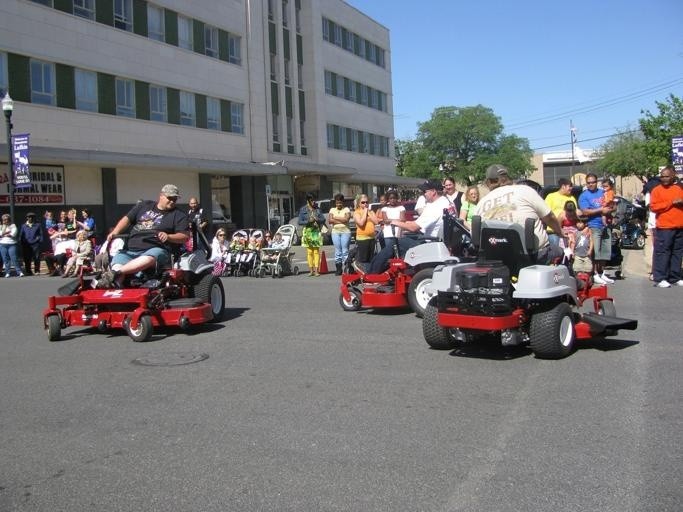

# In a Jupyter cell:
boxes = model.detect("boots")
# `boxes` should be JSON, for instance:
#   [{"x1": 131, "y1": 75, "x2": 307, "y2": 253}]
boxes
[{"x1": 335, "y1": 262, "x2": 343, "y2": 276}]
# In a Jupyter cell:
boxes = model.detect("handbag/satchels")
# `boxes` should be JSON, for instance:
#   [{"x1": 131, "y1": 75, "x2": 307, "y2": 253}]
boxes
[{"x1": 319, "y1": 222, "x2": 328, "y2": 234}]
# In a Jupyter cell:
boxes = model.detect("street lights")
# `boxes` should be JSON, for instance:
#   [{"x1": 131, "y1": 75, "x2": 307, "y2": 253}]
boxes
[{"x1": 1, "y1": 90, "x2": 17, "y2": 224}]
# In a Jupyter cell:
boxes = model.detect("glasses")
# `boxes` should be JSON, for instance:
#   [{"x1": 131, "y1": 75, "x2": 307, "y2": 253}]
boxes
[{"x1": 360, "y1": 201, "x2": 368, "y2": 204}]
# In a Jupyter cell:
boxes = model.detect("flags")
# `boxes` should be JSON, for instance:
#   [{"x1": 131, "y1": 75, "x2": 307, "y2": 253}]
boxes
[{"x1": 570, "y1": 126, "x2": 577, "y2": 143}]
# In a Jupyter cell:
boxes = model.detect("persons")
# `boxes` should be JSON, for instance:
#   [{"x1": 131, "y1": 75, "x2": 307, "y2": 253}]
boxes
[
  {"x1": 297, "y1": 192, "x2": 325, "y2": 277},
  {"x1": 645, "y1": 163, "x2": 683, "y2": 288},
  {"x1": 187, "y1": 198, "x2": 287, "y2": 276},
  {"x1": 632, "y1": 194, "x2": 640, "y2": 211},
  {"x1": 0, "y1": 207, "x2": 125, "y2": 278},
  {"x1": 579, "y1": 173, "x2": 617, "y2": 285},
  {"x1": 329, "y1": 176, "x2": 480, "y2": 275},
  {"x1": 471, "y1": 164, "x2": 586, "y2": 290},
  {"x1": 97, "y1": 184, "x2": 189, "y2": 289}
]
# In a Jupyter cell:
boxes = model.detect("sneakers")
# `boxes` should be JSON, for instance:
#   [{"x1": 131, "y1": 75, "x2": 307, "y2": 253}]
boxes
[
  {"x1": 593, "y1": 275, "x2": 608, "y2": 284},
  {"x1": 672, "y1": 279, "x2": 683, "y2": 286},
  {"x1": 308, "y1": 273, "x2": 314, "y2": 276},
  {"x1": 97, "y1": 270, "x2": 116, "y2": 288},
  {"x1": 652, "y1": 279, "x2": 671, "y2": 288},
  {"x1": 20, "y1": 273, "x2": 24, "y2": 276},
  {"x1": 315, "y1": 272, "x2": 320, "y2": 277},
  {"x1": 601, "y1": 273, "x2": 614, "y2": 284},
  {"x1": 5, "y1": 273, "x2": 10, "y2": 277},
  {"x1": 355, "y1": 261, "x2": 367, "y2": 275}
]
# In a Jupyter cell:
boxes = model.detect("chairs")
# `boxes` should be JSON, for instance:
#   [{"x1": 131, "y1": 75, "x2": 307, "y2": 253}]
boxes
[
  {"x1": 42, "y1": 245, "x2": 71, "y2": 276},
  {"x1": 73, "y1": 237, "x2": 96, "y2": 277}
]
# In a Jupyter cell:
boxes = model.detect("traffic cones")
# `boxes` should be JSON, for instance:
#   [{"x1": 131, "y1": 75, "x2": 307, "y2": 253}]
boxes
[{"x1": 319, "y1": 251, "x2": 330, "y2": 275}]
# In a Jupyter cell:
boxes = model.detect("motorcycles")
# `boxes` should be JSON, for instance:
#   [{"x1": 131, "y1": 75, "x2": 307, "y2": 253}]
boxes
[{"x1": 615, "y1": 207, "x2": 647, "y2": 249}]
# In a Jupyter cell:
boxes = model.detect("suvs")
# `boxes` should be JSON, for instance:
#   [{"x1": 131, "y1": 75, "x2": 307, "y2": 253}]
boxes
[
  {"x1": 289, "y1": 197, "x2": 357, "y2": 246},
  {"x1": 400, "y1": 200, "x2": 420, "y2": 234},
  {"x1": 211, "y1": 209, "x2": 231, "y2": 224}
]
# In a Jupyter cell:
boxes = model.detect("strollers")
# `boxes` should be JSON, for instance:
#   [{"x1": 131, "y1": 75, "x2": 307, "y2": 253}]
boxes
[
  {"x1": 253, "y1": 224, "x2": 299, "y2": 279},
  {"x1": 222, "y1": 228, "x2": 266, "y2": 281}
]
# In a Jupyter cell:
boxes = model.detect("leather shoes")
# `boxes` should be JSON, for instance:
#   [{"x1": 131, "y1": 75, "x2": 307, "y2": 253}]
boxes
[
  {"x1": 61, "y1": 273, "x2": 69, "y2": 278},
  {"x1": 70, "y1": 274, "x2": 77, "y2": 278}
]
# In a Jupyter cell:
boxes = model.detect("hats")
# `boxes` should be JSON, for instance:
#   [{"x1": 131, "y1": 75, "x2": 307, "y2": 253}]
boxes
[
  {"x1": 418, "y1": 179, "x2": 443, "y2": 191},
  {"x1": 161, "y1": 184, "x2": 181, "y2": 198},
  {"x1": 483, "y1": 164, "x2": 508, "y2": 184},
  {"x1": 26, "y1": 212, "x2": 35, "y2": 216}
]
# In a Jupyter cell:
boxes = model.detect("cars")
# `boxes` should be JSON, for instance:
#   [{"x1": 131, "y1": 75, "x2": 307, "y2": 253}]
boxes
[{"x1": 614, "y1": 196, "x2": 646, "y2": 220}]
